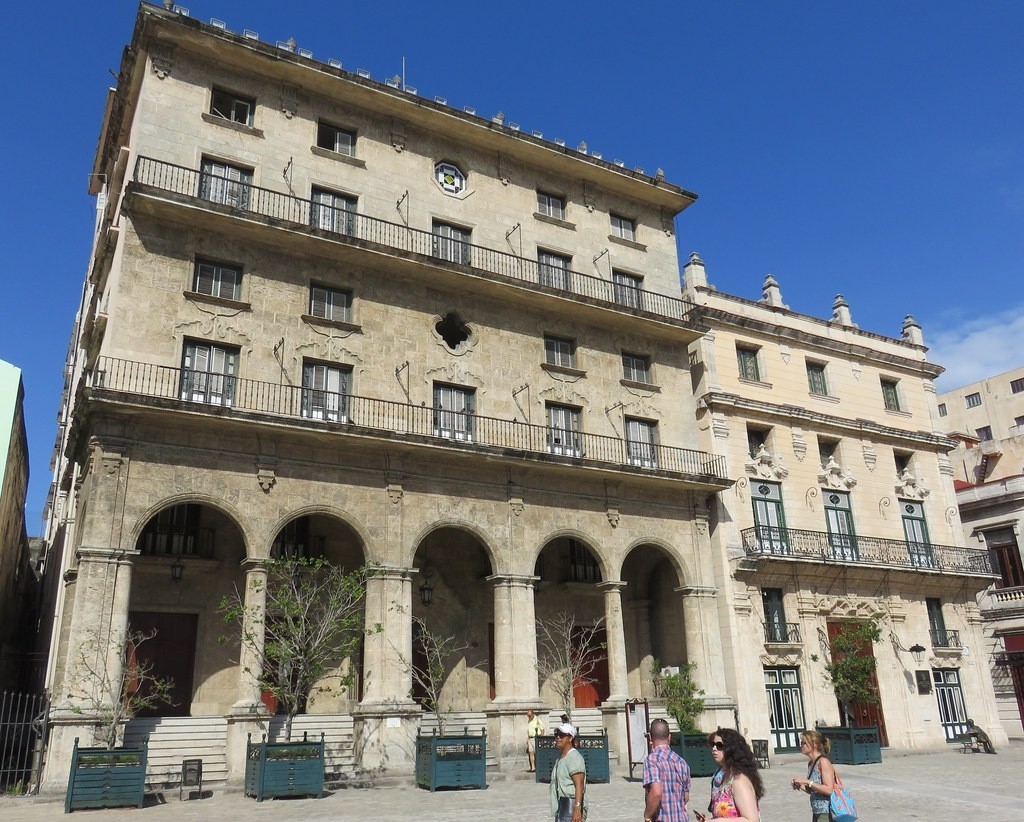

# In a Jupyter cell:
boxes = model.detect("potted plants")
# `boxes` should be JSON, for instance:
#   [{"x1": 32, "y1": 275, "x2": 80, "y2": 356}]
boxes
[
  {"x1": 813, "y1": 613, "x2": 889, "y2": 766},
  {"x1": 215, "y1": 548, "x2": 387, "y2": 804},
  {"x1": 53, "y1": 618, "x2": 185, "y2": 815},
  {"x1": 387, "y1": 610, "x2": 489, "y2": 792},
  {"x1": 646, "y1": 656, "x2": 721, "y2": 779},
  {"x1": 528, "y1": 605, "x2": 612, "y2": 785}
]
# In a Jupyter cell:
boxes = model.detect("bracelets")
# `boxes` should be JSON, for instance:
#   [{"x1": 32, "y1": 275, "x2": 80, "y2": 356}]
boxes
[
  {"x1": 573, "y1": 802, "x2": 581, "y2": 808},
  {"x1": 809, "y1": 781, "x2": 813, "y2": 788},
  {"x1": 644, "y1": 817, "x2": 652, "y2": 822}
]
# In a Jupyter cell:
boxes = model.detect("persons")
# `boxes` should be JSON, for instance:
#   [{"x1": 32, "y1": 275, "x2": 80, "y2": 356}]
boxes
[
  {"x1": 642, "y1": 718, "x2": 693, "y2": 822},
  {"x1": 560, "y1": 714, "x2": 567, "y2": 723},
  {"x1": 789, "y1": 730, "x2": 842, "y2": 822},
  {"x1": 548, "y1": 724, "x2": 588, "y2": 822},
  {"x1": 524, "y1": 709, "x2": 545, "y2": 773},
  {"x1": 696, "y1": 727, "x2": 766, "y2": 822},
  {"x1": 966, "y1": 719, "x2": 996, "y2": 754}
]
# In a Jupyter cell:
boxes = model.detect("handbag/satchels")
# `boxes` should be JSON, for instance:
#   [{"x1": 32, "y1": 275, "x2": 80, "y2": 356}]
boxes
[
  {"x1": 556, "y1": 760, "x2": 584, "y2": 822},
  {"x1": 819, "y1": 758, "x2": 858, "y2": 822}
]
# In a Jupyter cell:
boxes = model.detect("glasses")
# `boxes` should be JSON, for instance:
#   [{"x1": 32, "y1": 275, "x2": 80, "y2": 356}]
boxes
[
  {"x1": 711, "y1": 741, "x2": 725, "y2": 751},
  {"x1": 554, "y1": 733, "x2": 572, "y2": 738}
]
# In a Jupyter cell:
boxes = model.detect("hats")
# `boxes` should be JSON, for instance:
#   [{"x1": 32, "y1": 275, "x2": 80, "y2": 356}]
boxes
[{"x1": 555, "y1": 722, "x2": 577, "y2": 738}]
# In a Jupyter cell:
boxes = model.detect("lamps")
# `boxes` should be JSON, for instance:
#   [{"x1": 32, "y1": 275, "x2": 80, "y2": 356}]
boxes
[
  {"x1": 418, "y1": 538, "x2": 433, "y2": 608},
  {"x1": 169, "y1": 509, "x2": 188, "y2": 584},
  {"x1": 890, "y1": 630, "x2": 926, "y2": 667}
]
